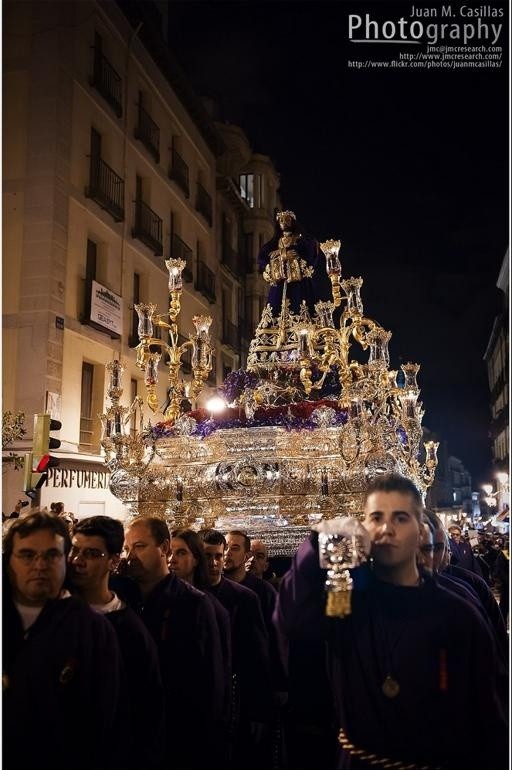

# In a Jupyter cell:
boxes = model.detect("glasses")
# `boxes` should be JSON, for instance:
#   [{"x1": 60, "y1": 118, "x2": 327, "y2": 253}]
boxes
[
  {"x1": 9, "y1": 548, "x2": 67, "y2": 565},
  {"x1": 66, "y1": 547, "x2": 112, "y2": 561}
]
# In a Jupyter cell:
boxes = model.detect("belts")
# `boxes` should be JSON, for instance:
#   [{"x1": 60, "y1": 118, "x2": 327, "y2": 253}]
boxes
[{"x1": 336, "y1": 726, "x2": 439, "y2": 770}]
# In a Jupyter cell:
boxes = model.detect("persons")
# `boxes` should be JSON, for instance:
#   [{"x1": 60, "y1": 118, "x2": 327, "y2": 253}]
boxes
[
  {"x1": 256, "y1": 206, "x2": 324, "y2": 323},
  {"x1": 2, "y1": 474, "x2": 509, "y2": 769}
]
